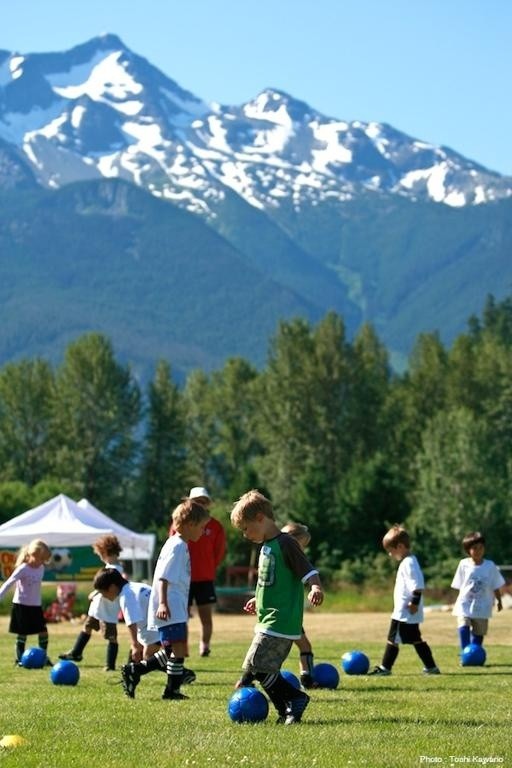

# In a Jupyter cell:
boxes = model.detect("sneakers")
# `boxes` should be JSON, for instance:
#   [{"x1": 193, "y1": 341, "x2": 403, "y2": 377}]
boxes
[
  {"x1": 161, "y1": 689, "x2": 188, "y2": 700},
  {"x1": 277, "y1": 710, "x2": 300, "y2": 724},
  {"x1": 418, "y1": 666, "x2": 440, "y2": 674},
  {"x1": 366, "y1": 663, "x2": 393, "y2": 676},
  {"x1": 181, "y1": 667, "x2": 195, "y2": 684},
  {"x1": 286, "y1": 692, "x2": 309, "y2": 728},
  {"x1": 121, "y1": 663, "x2": 138, "y2": 699},
  {"x1": 14, "y1": 655, "x2": 56, "y2": 668},
  {"x1": 59, "y1": 651, "x2": 84, "y2": 661},
  {"x1": 299, "y1": 672, "x2": 320, "y2": 689},
  {"x1": 101, "y1": 666, "x2": 117, "y2": 672}
]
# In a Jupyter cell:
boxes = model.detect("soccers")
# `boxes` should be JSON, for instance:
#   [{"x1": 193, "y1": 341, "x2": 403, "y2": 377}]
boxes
[
  {"x1": 343, "y1": 652, "x2": 369, "y2": 675},
  {"x1": 21, "y1": 648, "x2": 46, "y2": 668},
  {"x1": 462, "y1": 644, "x2": 486, "y2": 666},
  {"x1": 229, "y1": 688, "x2": 267, "y2": 722},
  {"x1": 51, "y1": 662, "x2": 79, "y2": 686},
  {"x1": 282, "y1": 671, "x2": 300, "y2": 690},
  {"x1": 313, "y1": 664, "x2": 339, "y2": 690}
]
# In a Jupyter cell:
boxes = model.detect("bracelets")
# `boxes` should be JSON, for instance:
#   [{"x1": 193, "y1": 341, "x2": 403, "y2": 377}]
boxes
[{"x1": 410, "y1": 592, "x2": 421, "y2": 606}]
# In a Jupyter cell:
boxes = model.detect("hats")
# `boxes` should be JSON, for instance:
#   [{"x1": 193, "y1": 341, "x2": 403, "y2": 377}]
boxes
[{"x1": 186, "y1": 486, "x2": 212, "y2": 502}]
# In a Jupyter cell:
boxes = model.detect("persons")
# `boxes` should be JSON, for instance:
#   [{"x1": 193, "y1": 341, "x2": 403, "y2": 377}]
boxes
[
  {"x1": 449, "y1": 531, "x2": 506, "y2": 649},
  {"x1": 229, "y1": 490, "x2": 324, "y2": 726},
  {"x1": 235, "y1": 522, "x2": 313, "y2": 689},
  {"x1": 58, "y1": 535, "x2": 126, "y2": 670},
  {"x1": 364, "y1": 522, "x2": 441, "y2": 674},
  {"x1": 95, "y1": 565, "x2": 195, "y2": 685},
  {"x1": 121, "y1": 496, "x2": 205, "y2": 698},
  {"x1": 0, "y1": 539, "x2": 54, "y2": 669},
  {"x1": 168, "y1": 486, "x2": 226, "y2": 658}
]
